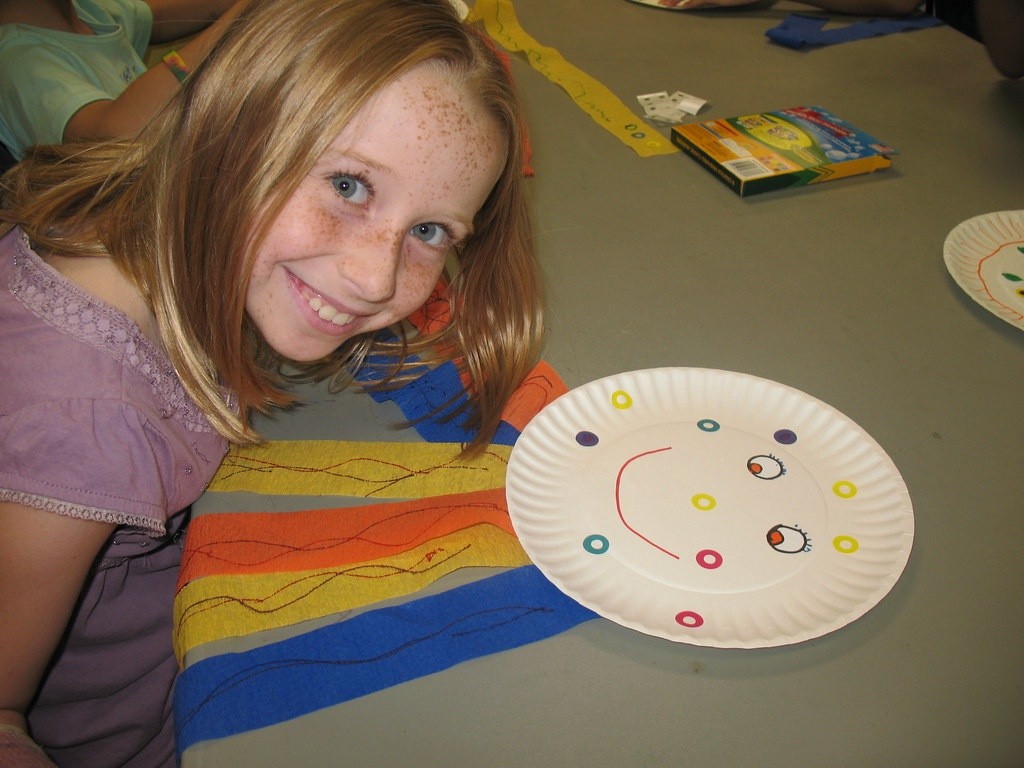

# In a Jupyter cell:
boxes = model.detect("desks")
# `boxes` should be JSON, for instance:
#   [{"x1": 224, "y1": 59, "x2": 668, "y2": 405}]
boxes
[{"x1": 171, "y1": 2, "x2": 1024, "y2": 768}]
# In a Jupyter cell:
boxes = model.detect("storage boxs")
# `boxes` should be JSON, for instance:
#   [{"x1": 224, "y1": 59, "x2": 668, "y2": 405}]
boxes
[{"x1": 671, "y1": 106, "x2": 901, "y2": 199}]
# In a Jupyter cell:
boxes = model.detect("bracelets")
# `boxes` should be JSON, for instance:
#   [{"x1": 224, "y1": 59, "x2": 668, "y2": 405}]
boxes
[{"x1": 163, "y1": 50, "x2": 192, "y2": 83}]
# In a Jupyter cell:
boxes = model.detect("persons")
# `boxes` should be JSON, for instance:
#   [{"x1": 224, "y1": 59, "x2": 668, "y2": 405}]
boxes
[
  {"x1": 0, "y1": 1, "x2": 547, "y2": 768},
  {"x1": 0, "y1": 0, "x2": 251, "y2": 162}
]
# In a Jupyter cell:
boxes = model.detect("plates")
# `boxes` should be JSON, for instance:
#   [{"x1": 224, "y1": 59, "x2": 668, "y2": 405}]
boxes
[
  {"x1": 507, "y1": 368, "x2": 914, "y2": 649},
  {"x1": 942, "y1": 209, "x2": 1024, "y2": 336}
]
